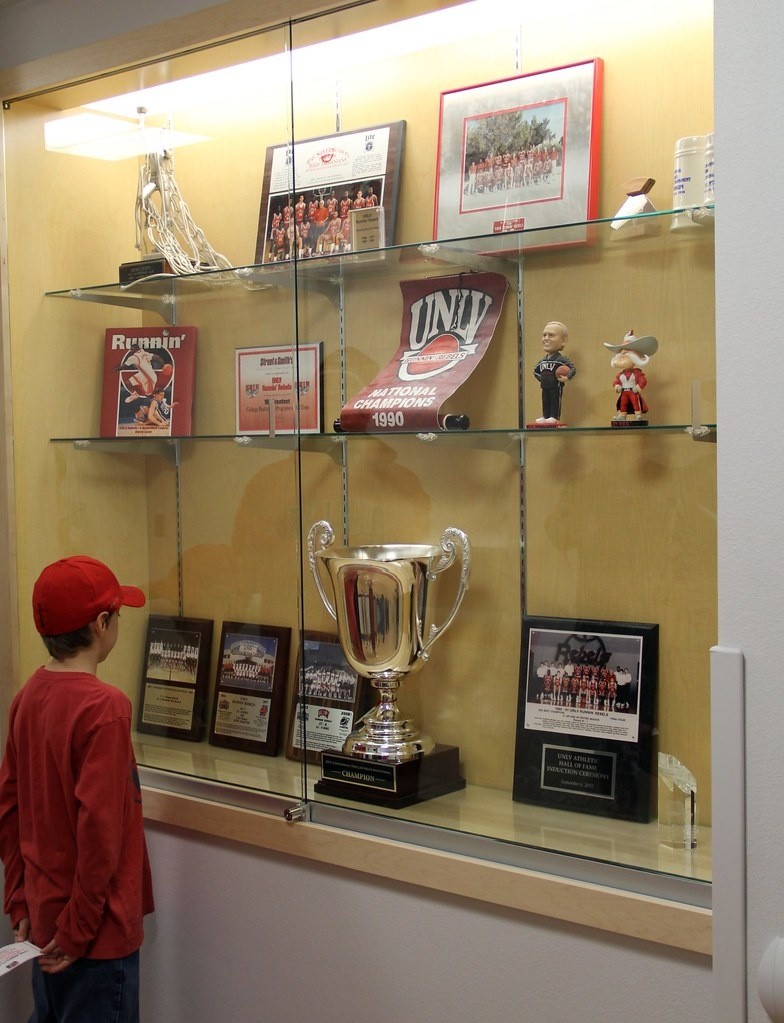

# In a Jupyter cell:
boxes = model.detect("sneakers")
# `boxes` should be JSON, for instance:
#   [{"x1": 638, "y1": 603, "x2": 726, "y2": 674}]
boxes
[
  {"x1": 536, "y1": 417, "x2": 545, "y2": 423},
  {"x1": 545, "y1": 417, "x2": 558, "y2": 423},
  {"x1": 125, "y1": 391, "x2": 140, "y2": 403}
]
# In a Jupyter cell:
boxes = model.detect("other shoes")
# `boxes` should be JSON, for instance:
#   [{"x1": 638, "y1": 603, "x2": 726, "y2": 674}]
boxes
[
  {"x1": 635, "y1": 414, "x2": 642, "y2": 421},
  {"x1": 612, "y1": 416, "x2": 626, "y2": 420}
]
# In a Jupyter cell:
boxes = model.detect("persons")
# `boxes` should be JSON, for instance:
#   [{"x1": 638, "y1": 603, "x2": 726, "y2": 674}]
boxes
[
  {"x1": 602, "y1": 327, "x2": 661, "y2": 427},
  {"x1": 3, "y1": 553, "x2": 159, "y2": 1023},
  {"x1": 533, "y1": 654, "x2": 636, "y2": 711},
  {"x1": 132, "y1": 398, "x2": 157, "y2": 426},
  {"x1": 299, "y1": 659, "x2": 358, "y2": 702},
  {"x1": 142, "y1": 633, "x2": 204, "y2": 680},
  {"x1": 147, "y1": 386, "x2": 172, "y2": 429},
  {"x1": 464, "y1": 137, "x2": 557, "y2": 196},
  {"x1": 267, "y1": 186, "x2": 376, "y2": 270},
  {"x1": 113, "y1": 345, "x2": 164, "y2": 398},
  {"x1": 219, "y1": 658, "x2": 273, "y2": 685},
  {"x1": 527, "y1": 320, "x2": 578, "y2": 428}
]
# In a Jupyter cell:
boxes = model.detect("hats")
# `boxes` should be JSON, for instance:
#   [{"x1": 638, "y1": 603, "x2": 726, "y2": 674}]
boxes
[
  {"x1": 32, "y1": 555, "x2": 146, "y2": 635},
  {"x1": 604, "y1": 329, "x2": 658, "y2": 357}
]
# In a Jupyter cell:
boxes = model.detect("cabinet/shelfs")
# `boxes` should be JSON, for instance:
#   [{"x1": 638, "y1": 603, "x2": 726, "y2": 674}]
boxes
[{"x1": 1, "y1": 5, "x2": 726, "y2": 958}]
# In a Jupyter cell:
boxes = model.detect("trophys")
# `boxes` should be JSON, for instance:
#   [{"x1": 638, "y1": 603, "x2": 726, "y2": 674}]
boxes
[{"x1": 304, "y1": 517, "x2": 472, "y2": 760}]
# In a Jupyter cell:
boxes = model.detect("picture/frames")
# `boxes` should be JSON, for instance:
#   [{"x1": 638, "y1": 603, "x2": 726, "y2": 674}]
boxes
[
  {"x1": 234, "y1": 341, "x2": 326, "y2": 439},
  {"x1": 254, "y1": 118, "x2": 405, "y2": 278},
  {"x1": 286, "y1": 627, "x2": 366, "y2": 767},
  {"x1": 209, "y1": 621, "x2": 290, "y2": 758},
  {"x1": 429, "y1": 53, "x2": 606, "y2": 263},
  {"x1": 137, "y1": 612, "x2": 214, "y2": 746},
  {"x1": 512, "y1": 614, "x2": 661, "y2": 826}
]
